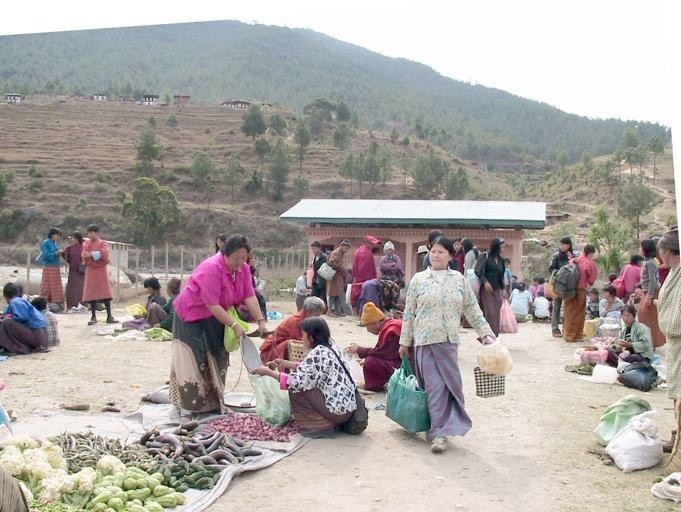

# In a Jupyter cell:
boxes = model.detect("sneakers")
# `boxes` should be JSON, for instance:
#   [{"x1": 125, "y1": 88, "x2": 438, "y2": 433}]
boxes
[
  {"x1": 552, "y1": 330, "x2": 562, "y2": 337},
  {"x1": 431, "y1": 434, "x2": 448, "y2": 454}
]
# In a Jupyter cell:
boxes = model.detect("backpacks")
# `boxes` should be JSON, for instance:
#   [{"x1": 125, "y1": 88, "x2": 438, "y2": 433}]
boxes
[{"x1": 551, "y1": 263, "x2": 580, "y2": 302}]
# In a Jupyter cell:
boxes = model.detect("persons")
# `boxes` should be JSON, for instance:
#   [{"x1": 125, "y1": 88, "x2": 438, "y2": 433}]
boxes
[
  {"x1": 39, "y1": 227, "x2": 65, "y2": 312},
  {"x1": 124, "y1": 231, "x2": 681, "y2": 452},
  {"x1": 0, "y1": 283, "x2": 60, "y2": 355},
  {"x1": 64, "y1": 231, "x2": 104, "y2": 311},
  {"x1": 82, "y1": 224, "x2": 119, "y2": 325}
]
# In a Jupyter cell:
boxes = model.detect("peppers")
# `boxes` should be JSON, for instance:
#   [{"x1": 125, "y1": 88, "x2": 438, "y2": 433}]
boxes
[{"x1": 49, "y1": 431, "x2": 148, "y2": 472}]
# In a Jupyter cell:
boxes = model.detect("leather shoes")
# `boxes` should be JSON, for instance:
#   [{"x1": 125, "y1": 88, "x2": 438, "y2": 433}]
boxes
[
  {"x1": 88, "y1": 318, "x2": 98, "y2": 325},
  {"x1": 107, "y1": 318, "x2": 121, "y2": 323}
]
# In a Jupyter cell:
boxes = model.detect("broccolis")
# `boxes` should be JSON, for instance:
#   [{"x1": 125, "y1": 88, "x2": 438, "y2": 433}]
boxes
[{"x1": 1, "y1": 435, "x2": 126, "y2": 509}]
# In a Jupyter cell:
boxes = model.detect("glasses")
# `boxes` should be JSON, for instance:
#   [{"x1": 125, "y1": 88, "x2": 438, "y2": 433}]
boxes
[{"x1": 216, "y1": 240, "x2": 225, "y2": 243}]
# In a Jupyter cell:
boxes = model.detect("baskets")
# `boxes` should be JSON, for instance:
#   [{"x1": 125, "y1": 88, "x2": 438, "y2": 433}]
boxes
[{"x1": 289, "y1": 339, "x2": 306, "y2": 373}]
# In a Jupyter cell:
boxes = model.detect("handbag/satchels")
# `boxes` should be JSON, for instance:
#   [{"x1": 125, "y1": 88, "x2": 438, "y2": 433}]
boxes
[
  {"x1": 341, "y1": 395, "x2": 370, "y2": 434},
  {"x1": 466, "y1": 268, "x2": 483, "y2": 302},
  {"x1": 473, "y1": 366, "x2": 506, "y2": 399},
  {"x1": 543, "y1": 281, "x2": 561, "y2": 299},
  {"x1": 306, "y1": 267, "x2": 316, "y2": 289},
  {"x1": 384, "y1": 372, "x2": 431, "y2": 434},
  {"x1": 317, "y1": 262, "x2": 338, "y2": 282}
]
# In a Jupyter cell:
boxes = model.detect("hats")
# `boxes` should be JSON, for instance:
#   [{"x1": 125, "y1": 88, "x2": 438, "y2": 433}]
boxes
[
  {"x1": 657, "y1": 225, "x2": 678, "y2": 251},
  {"x1": 360, "y1": 302, "x2": 386, "y2": 327},
  {"x1": 383, "y1": 240, "x2": 396, "y2": 253},
  {"x1": 167, "y1": 276, "x2": 181, "y2": 289},
  {"x1": 417, "y1": 245, "x2": 430, "y2": 255},
  {"x1": 339, "y1": 238, "x2": 352, "y2": 249}
]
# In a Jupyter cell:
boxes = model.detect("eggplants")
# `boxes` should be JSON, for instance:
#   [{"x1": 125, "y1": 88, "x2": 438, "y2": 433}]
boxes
[{"x1": 133, "y1": 420, "x2": 263, "y2": 463}]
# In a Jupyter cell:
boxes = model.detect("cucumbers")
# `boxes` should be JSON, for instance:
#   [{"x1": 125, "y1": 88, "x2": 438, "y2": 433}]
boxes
[{"x1": 152, "y1": 456, "x2": 224, "y2": 492}]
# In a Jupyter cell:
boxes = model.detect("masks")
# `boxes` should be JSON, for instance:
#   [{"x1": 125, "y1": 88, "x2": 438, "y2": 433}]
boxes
[{"x1": 372, "y1": 247, "x2": 378, "y2": 252}]
